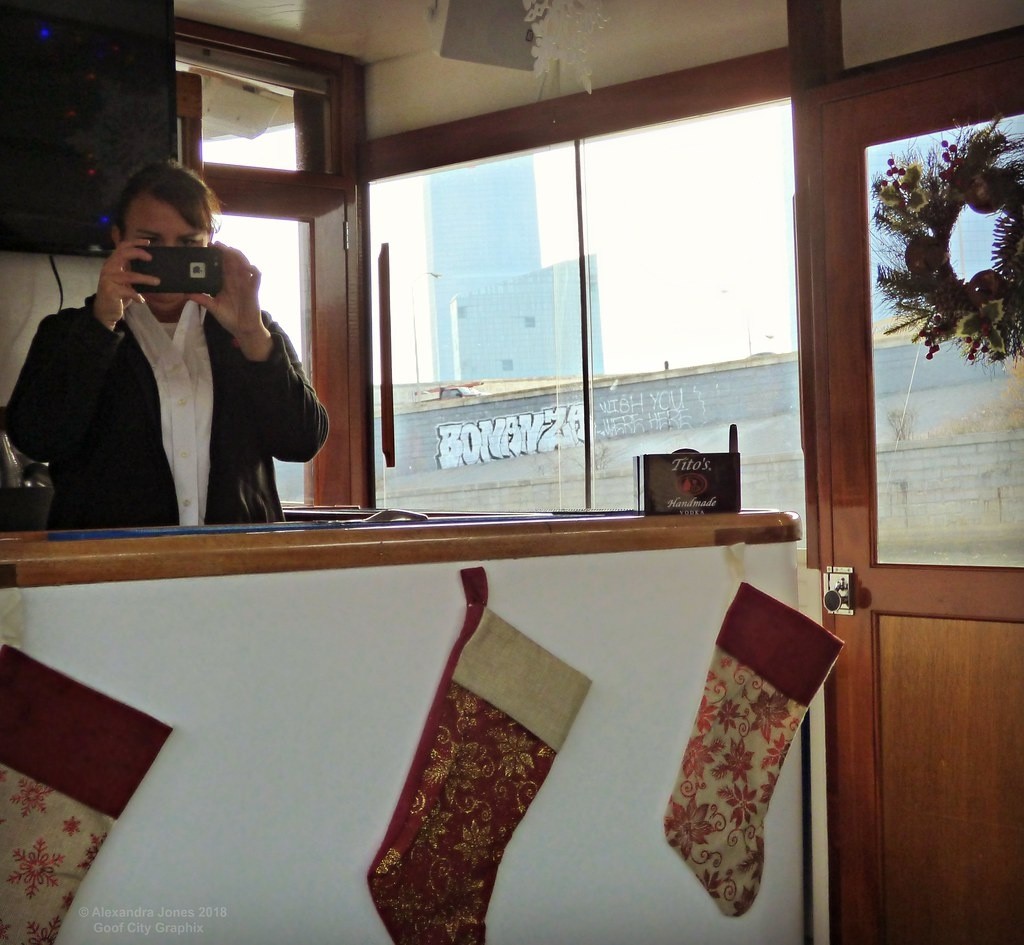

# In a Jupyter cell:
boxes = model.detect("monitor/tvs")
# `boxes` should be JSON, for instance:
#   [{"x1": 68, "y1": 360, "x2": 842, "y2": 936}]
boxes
[{"x1": 0, "y1": 0, "x2": 178, "y2": 259}]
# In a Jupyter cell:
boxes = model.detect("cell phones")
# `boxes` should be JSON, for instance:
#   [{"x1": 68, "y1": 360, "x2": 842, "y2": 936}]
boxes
[{"x1": 131, "y1": 244, "x2": 223, "y2": 293}]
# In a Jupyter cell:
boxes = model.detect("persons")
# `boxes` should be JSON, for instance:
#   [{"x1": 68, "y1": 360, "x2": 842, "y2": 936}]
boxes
[{"x1": 4, "y1": 160, "x2": 330, "y2": 531}]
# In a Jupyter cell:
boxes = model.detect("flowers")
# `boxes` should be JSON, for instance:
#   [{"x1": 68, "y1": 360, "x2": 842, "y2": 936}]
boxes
[{"x1": 872, "y1": 108, "x2": 1024, "y2": 381}]
